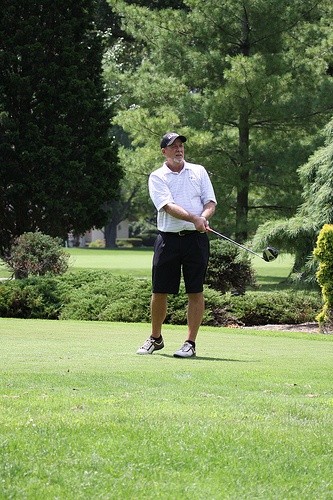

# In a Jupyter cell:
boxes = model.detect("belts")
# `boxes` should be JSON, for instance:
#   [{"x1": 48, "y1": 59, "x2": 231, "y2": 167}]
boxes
[{"x1": 165, "y1": 230, "x2": 200, "y2": 237}]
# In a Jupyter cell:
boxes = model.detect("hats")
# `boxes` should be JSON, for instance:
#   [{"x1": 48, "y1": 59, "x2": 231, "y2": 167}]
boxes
[{"x1": 160, "y1": 132, "x2": 187, "y2": 149}]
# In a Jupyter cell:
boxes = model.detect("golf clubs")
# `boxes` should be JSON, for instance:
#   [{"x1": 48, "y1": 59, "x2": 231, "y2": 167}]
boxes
[{"x1": 206, "y1": 227, "x2": 279, "y2": 262}]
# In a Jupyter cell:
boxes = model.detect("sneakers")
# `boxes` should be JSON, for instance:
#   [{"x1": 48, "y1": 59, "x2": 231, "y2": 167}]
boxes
[
  {"x1": 136, "y1": 335, "x2": 164, "y2": 355},
  {"x1": 173, "y1": 342, "x2": 196, "y2": 358}
]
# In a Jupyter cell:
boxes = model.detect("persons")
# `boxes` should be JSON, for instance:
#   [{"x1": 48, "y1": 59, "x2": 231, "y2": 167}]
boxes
[{"x1": 137, "y1": 133, "x2": 217, "y2": 357}]
